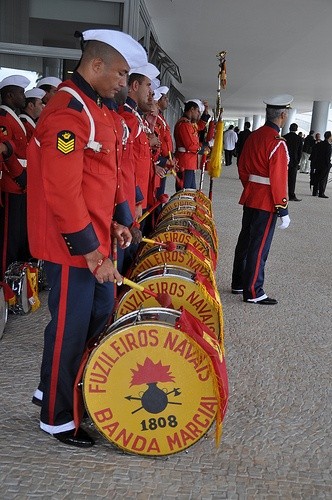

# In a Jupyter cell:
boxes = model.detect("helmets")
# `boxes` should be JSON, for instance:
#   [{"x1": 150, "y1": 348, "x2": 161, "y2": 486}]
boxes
[{"x1": 184, "y1": 98, "x2": 205, "y2": 112}]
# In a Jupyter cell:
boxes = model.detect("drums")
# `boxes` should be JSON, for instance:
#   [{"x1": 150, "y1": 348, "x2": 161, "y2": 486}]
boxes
[
  {"x1": 141, "y1": 227, "x2": 220, "y2": 268},
  {"x1": 80, "y1": 307, "x2": 227, "y2": 457},
  {"x1": 126, "y1": 243, "x2": 220, "y2": 299},
  {"x1": 154, "y1": 187, "x2": 219, "y2": 254},
  {"x1": 114, "y1": 263, "x2": 225, "y2": 343},
  {"x1": 0, "y1": 284, "x2": 11, "y2": 339},
  {"x1": 5, "y1": 260, "x2": 39, "y2": 317}
]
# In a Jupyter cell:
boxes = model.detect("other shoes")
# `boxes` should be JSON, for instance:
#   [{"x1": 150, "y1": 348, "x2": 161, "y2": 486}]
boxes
[
  {"x1": 300, "y1": 171, "x2": 309, "y2": 174},
  {"x1": 31, "y1": 395, "x2": 42, "y2": 407},
  {"x1": 312, "y1": 192, "x2": 319, "y2": 196},
  {"x1": 225, "y1": 162, "x2": 232, "y2": 166},
  {"x1": 318, "y1": 194, "x2": 329, "y2": 198},
  {"x1": 231, "y1": 289, "x2": 244, "y2": 294},
  {"x1": 289, "y1": 198, "x2": 302, "y2": 201},
  {"x1": 40, "y1": 424, "x2": 93, "y2": 447},
  {"x1": 243, "y1": 297, "x2": 278, "y2": 304}
]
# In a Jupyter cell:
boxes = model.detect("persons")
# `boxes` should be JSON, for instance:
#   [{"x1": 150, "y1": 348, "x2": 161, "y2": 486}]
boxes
[
  {"x1": 282, "y1": 123, "x2": 303, "y2": 201},
  {"x1": 26, "y1": 29, "x2": 148, "y2": 448},
  {"x1": 97, "y1": 62, "x2": 173, "y2": 299},
  {"x1": 298, "y1": 130, "x2": 322, "y2": 174},
  {"x1": 223, "y1": 125, "x2": 240, "y2": 166},
  {"x1": 229, "y1": 94, "x2": 294, "y2": 305},
  {"x1": 0, "y1": 74, "x2": 61, "y2": 291},
  {"x1": 311, "y1": 131, "x2": 332, "y2": 198},
  {"x1": 234, "y1": 122, "x2": 253, "y2": 166},
  {"x1": 173, "y1": 98, "x2": 215, "y2": 192}
]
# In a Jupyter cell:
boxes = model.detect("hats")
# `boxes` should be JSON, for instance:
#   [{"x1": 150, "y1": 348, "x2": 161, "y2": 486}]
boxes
[
  {"x1": 24, "y1": 88, "x2": 46, "y2": 99},
  {"x1": 154, "y1": 86, "x2": 169, "y2": 101},
  {"x1": 128, "y1": 63, "x2": 160, "y2": 80},
  {"x1": 82, "y1": 29, "x2": 148, "y2": 69},
  {"x1": 0, "y1": 75, "x2": 30, "y2": 89},
  {"x1": 150, "y1": 78, "x2": 160, "y2": 91},
  {"x1": 263, "y1": 94, "x2": 294, "y2": 109},
  {"x1": 36, "y1": 77, "x2": 62, "y2": 88}
]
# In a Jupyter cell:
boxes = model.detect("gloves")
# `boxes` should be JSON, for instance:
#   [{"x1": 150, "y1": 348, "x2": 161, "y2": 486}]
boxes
[{"x1": 278, "y1": 215, "x2": 290, "y2": 229}]
